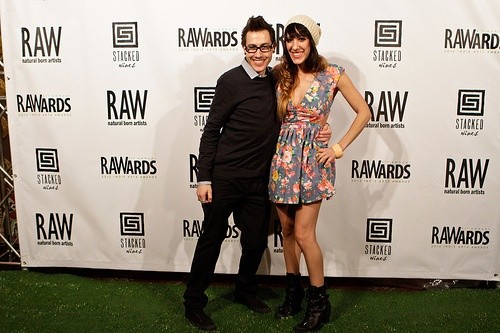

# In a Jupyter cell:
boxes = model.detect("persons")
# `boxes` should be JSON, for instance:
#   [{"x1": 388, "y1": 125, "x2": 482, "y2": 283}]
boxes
[
  {"x1": 180, "y1": 12, "x2": 331, "y2": 333},
  {"x1": 269, "y1": 14, "x2": 372, "y2": 329}
]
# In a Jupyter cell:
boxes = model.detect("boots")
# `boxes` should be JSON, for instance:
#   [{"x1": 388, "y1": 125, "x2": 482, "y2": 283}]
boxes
[
  {"x1": 293, "y1": 285, "x2": 331, "y2": 333},
  {"x1": 275, "y1": 273, "x2": 304, "y2": 319}
]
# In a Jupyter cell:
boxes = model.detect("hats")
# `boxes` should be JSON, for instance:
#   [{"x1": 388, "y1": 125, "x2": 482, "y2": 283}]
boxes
[{"x1": 284, "y1": 15, "x2": 321, "y2": 48}]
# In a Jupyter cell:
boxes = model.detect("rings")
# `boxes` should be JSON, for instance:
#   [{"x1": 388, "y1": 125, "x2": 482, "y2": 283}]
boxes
[{"x1": 198, "y1": 198, "x2": 206, "y2": 203}]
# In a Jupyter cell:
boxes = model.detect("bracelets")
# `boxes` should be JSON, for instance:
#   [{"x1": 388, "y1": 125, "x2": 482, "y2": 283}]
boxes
[{"x1": 331, "y1": 143, "x2": 344, "y2": 158}]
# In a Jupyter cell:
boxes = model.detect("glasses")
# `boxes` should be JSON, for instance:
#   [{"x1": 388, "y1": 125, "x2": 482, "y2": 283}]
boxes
[{"x1": 245, "y1": 41, "x2": 274, "y2": 53}]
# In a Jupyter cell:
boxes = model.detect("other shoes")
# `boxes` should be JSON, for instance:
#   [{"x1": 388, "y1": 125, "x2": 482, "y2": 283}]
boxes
[
  {"x1": 235, "y1": 297, "x2": 270, "y2": 313},
  {"x1": 184, "y1": 308, "x2": 215, "y2": 331}
]
